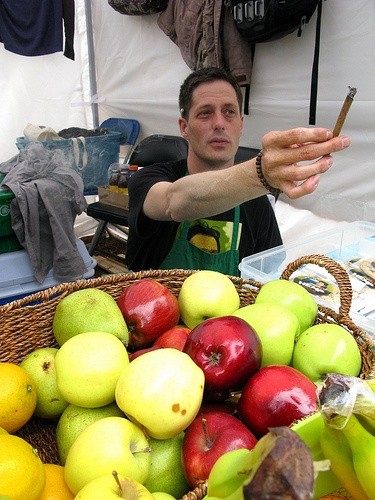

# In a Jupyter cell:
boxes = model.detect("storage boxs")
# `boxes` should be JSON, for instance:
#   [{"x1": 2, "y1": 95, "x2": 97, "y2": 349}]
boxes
[
  {"x1": 238, "y1": 221, "x2": 375, "y2": 342},
  {"x1": 0, "y1": 131, "x2": 129, "y2": 308}
]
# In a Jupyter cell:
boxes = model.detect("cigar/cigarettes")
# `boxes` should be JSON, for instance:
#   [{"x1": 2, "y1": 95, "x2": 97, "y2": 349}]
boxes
[{"x1": 333, "y1": 86, "x2": 358, "y2": 138}]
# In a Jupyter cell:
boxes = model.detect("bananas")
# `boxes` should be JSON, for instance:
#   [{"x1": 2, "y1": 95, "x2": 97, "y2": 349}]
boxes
[{"x1": 199, "y1": 369, "x2": 375, "y2": 500}]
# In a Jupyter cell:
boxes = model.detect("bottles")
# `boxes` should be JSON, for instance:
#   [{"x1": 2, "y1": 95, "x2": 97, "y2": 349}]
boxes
[{"x1": 109, "y1": 163, "x2": 140, "y2": 196}]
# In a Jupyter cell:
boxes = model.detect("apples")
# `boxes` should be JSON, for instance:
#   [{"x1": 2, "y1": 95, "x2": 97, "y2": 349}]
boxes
[{"x1": 0, "y1": 268, "x2": 361, "y2": 500}]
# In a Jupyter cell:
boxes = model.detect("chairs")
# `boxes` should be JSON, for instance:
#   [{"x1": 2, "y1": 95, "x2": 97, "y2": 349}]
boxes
[{"x1": 82, "y1": 117, "x2": 279, "y2": 283}]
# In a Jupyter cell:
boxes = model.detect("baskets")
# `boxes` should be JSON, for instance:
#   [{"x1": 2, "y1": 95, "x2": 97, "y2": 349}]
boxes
[{"x1": 0, "y1": 253, "x2": 375, "y2": 500}]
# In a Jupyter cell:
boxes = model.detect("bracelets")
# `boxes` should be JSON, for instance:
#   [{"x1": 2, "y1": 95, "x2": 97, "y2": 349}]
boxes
[{"x1": 256, "y1": 151, "x2": 283, "y2": 203}]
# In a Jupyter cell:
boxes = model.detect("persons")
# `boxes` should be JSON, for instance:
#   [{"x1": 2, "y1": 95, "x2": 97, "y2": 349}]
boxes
[{"x1": 125, "y1": 66, "x2": 351, "y2": 286}]
